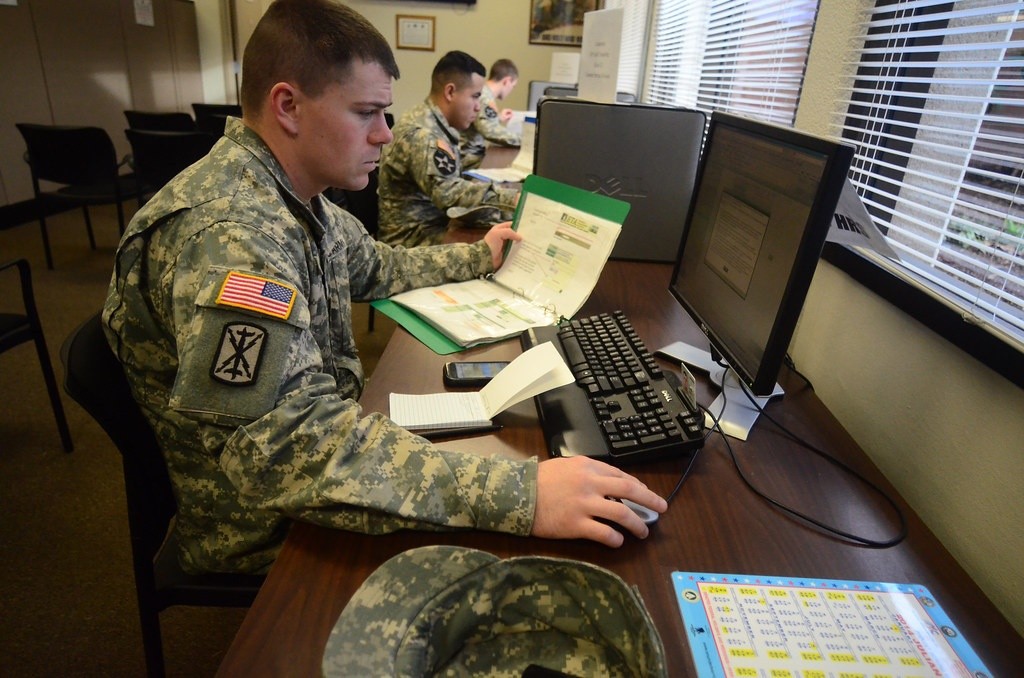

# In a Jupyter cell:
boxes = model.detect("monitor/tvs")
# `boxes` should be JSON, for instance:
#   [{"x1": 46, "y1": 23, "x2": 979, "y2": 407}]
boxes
[{"x1": 653, "y1": 109, "x2": 857, "y2": 439}]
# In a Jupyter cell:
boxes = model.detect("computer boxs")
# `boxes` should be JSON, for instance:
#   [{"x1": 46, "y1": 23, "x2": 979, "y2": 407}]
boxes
[{"x1": 526, "y1": 80, "x2": 705, "y2": 262}]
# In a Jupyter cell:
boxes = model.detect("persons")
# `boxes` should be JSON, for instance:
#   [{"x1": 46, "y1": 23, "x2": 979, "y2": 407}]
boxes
[
  {"x1": 99, "y1": 0, "x2": 668, "y2": 578},
  {"x1": 457, "y1": 59, "x2": 522, "y2": 171},
  {"x1": 375, "y1": 49, "x2": 523, "y2": 248}
]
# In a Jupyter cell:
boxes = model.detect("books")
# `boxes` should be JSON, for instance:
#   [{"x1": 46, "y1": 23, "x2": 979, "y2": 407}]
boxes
[{"x1": 389, "y1": 341, "x2": 576, "y2": 431}]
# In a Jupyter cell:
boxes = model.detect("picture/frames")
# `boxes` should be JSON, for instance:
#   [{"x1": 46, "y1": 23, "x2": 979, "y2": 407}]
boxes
[
  {"x1": 529, "y1": 0, "x2": 599, "y2": 47},
  {"x1": 396, "y1": 14, "x2": 436, "y2": 52}
]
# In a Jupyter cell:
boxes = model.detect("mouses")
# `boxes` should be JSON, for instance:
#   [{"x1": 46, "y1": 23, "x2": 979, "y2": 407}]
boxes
[{"x1": 592, "y1": 496, "x2": 659, "y2": 526}]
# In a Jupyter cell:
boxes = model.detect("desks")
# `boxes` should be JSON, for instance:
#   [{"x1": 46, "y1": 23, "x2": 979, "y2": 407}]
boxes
[{"x1": 215, "y1": 145, "x2": 1024, "y2": 678}]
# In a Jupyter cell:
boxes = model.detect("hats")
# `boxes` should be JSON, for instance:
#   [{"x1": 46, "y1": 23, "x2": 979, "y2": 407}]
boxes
[
  {"x1": 446, "y1": 202, "x2": 514, "y2": 229},
  {"x1": 322, "y1": 545, "x2": 668, "y2": 678}
]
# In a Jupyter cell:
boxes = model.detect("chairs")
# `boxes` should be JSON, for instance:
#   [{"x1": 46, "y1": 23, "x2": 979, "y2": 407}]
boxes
[
  {"x1": 59, "y1": 310, "x2": 265, "y2": 678},
  {"x1": 124, "y1": 101, "x2": 241, "y2": 208},
  {"x1": 16, "y1": 122, "x2": 142, "y2": 269},
  {"x1": 0, "y1": 258, "x2": 76, "y2": 451}
]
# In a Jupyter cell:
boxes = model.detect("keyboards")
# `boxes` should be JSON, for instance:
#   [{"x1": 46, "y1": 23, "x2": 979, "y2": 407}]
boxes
[{"x1": 520, "y1": 310, "x2": 704, "y2": 466}]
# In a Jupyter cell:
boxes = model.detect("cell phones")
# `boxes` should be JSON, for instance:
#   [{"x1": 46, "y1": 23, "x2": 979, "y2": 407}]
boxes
[{"x1": 443, "y1": 360, "x2": 511, "y2": 384}]
